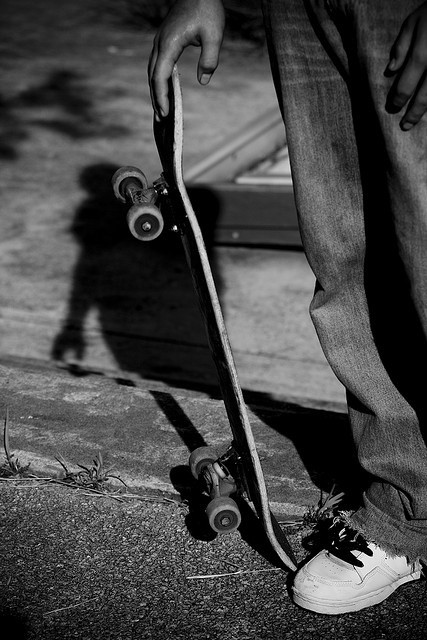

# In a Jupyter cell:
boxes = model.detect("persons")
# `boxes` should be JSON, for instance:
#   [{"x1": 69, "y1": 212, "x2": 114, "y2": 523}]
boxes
[{"x1": 146, "y1": 0, "x2": 427, "y2": 617}]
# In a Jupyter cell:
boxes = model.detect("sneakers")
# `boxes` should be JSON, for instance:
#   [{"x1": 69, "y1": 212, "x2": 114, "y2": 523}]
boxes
[{"x1": 290, "y1": 523, "x2": 425, "y2": 615}]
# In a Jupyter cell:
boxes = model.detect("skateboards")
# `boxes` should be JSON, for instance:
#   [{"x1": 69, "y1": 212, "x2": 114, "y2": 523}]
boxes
[{"x1": 111, "y1": 61, "x2": 299, "y2": 574}]
